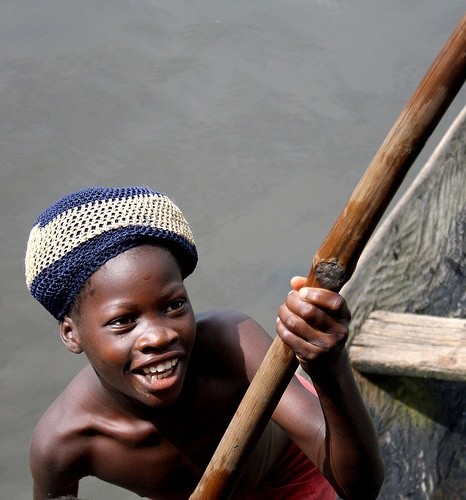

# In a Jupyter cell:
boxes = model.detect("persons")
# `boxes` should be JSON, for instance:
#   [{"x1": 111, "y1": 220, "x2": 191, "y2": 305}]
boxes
[{"x1": 24, "y1": 186, "x2": 384, "y2": 499}]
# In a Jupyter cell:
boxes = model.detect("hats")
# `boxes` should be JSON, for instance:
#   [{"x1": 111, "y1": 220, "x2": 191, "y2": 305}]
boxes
[{"x1": 26, "y1": 186, "x2": 198, "y2": 320}]
